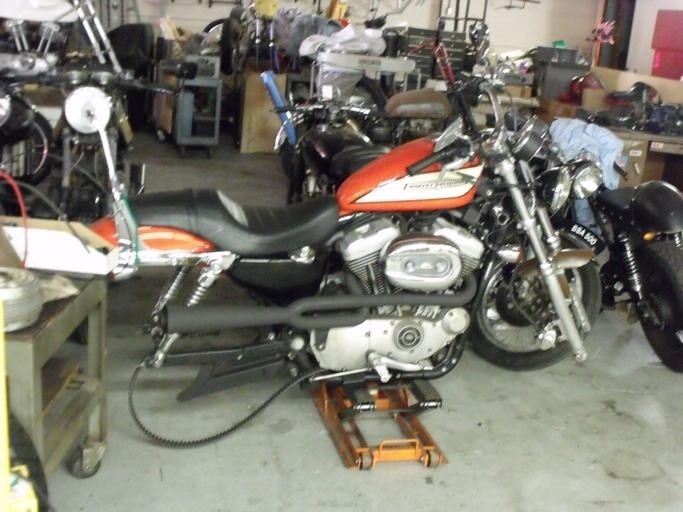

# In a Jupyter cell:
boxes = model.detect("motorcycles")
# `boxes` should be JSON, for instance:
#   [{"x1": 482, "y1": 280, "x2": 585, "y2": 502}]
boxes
[
  {"x1": 62, "y1": 26, "x2": 606, "y2": 396},
  {"x1": 0, "y1": 0, "x2": 681, "y2": 373}
]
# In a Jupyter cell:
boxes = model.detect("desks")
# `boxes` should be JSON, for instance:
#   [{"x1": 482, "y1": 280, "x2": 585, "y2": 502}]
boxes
[
  {"x1": 582, "y1": 67, "x2": 683, "y2": 187},
  {"x1": 237, "y1": 74, "x2": 286, "y2": 155}
]
